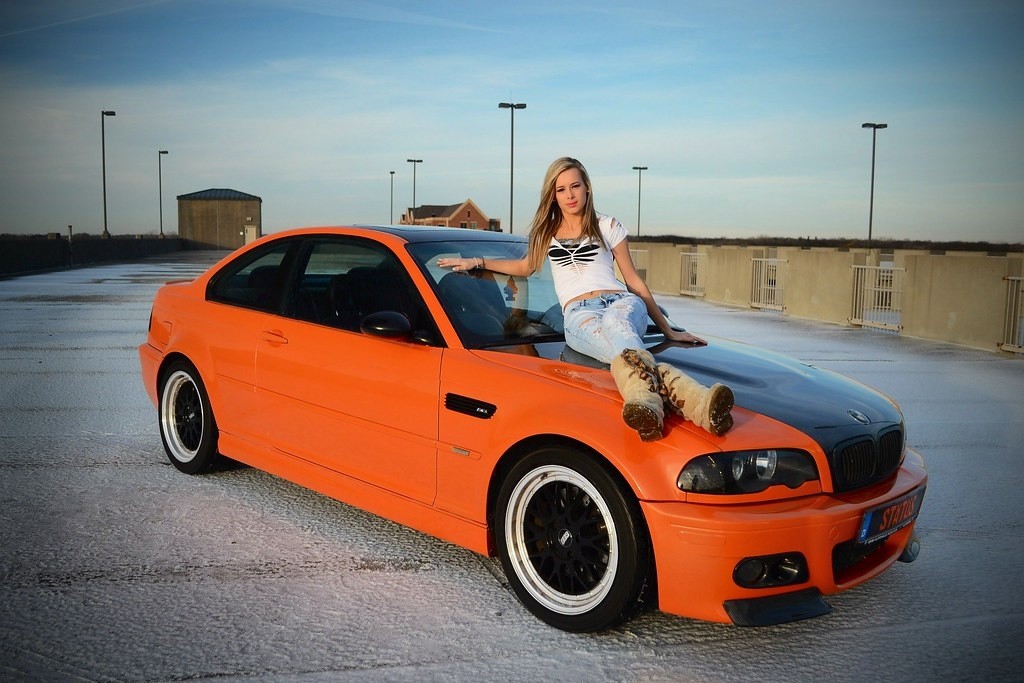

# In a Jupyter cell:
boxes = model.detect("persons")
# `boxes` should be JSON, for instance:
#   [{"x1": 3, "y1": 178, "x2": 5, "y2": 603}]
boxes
[{"x1": 436, "y1": 156, "x2": 734, "y2": 442}]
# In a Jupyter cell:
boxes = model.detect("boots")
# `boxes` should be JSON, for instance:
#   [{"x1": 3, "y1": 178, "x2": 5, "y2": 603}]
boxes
[
  {"x1": 656, "y1": 362, "x2": 734, "y2": 436},
  {"x1": 610, "y1": 348, "x2": 664, "y2": 442}
]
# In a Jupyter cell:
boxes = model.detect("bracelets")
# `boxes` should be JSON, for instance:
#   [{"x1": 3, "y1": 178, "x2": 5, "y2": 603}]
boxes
[
  {"x1": 480, "y1": 257, "x2": 486, "y2": 269},
  {"x1": 473, "y1": 257, "x2": 478, "y2": 269}
]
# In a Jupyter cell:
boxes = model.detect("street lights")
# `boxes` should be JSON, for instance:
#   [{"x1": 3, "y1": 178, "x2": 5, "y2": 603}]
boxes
[
  {"x1": 498, "y1": 103, "x2": 526, "y2": 234},
  {"x1": 862, "y1": 122, "x2": 887, "y2": 248},
  {"x1": 633, "y1": 166, "x2": 648, "y2": 242},
  {"x1": 159, "y1": 150, "x2": 168, "y2": 233},
  {"x1": 390, "y1": 171, "x2": 395, "y2": 225},
  {"x1": 407, "y1": 159, "x2": 423, "y2": 225},
  {"x1": 101, "y1": 110, "x2": 116, "y2": 231}
]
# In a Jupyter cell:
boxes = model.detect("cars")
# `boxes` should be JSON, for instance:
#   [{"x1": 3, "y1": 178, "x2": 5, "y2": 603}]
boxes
[{"x1": 139, "y1": 226, "x2": 927, "y2": 633}]
